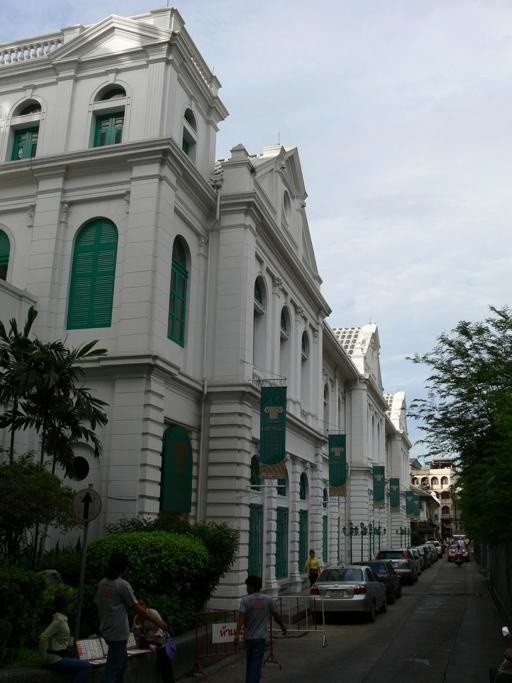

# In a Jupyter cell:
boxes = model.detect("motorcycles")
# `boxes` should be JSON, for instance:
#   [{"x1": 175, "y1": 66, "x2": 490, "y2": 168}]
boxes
[{"x1": 489, "y1": 625, "x2": 512, "y2": 682}]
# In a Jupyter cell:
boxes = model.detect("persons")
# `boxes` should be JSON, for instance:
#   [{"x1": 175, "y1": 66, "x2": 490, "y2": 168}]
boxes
[
  {"x1": 454, "y1": 544, "x2": 462, "y2": 552},
  {"x1": 130, "y1": 595, "x2": 178, "y2": 683},
  {"x1": 92, "y1": 551, "x2": 170, "y2": 682},
  {"x1": 493, "y1": 647, "x2": 512, "y2": 682},
  {"x1": 38, "y1": 591, "x2": 93, "y2": 682},
  {"x1": 232, "y1": 573, "x2": 288, "y2": 683},
  {"x1": 302, "y1": 548, "x2": 321, "y2": 589}
]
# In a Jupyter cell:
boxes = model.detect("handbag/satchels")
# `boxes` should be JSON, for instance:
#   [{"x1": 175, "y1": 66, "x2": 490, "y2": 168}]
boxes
[
  {"x1": 57, "y1": 649, "x2": 72, "y2": 657},
  {"x1": 310, "y1": 569, "x2": 318, "y2": 576}
]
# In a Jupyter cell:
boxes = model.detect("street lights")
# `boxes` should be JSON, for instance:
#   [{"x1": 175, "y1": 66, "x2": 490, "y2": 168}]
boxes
[
  {"x1": 342, "y1": 521, "x2": 386, "y2": 563},
  {"x1": 397, "y1": 527, "x2": 406, "y2": 547}
]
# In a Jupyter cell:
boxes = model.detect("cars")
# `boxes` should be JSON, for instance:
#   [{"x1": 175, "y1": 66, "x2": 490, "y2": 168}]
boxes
[
  {"x1": 375, "y1": 548, "x2": 417, "y2": 585},
  {"x1": 351, "y1": 560, "x2": 401, "y2": 604},
  {"x1": 310, "y1": 564, "x2": 386, "y2": 621},
  {"x1": 448, "y1": 543, "x2": 469, "y2": 562},
  {"x1": 408, "y1": 539, "x2": 442, "y2": 573}
]
